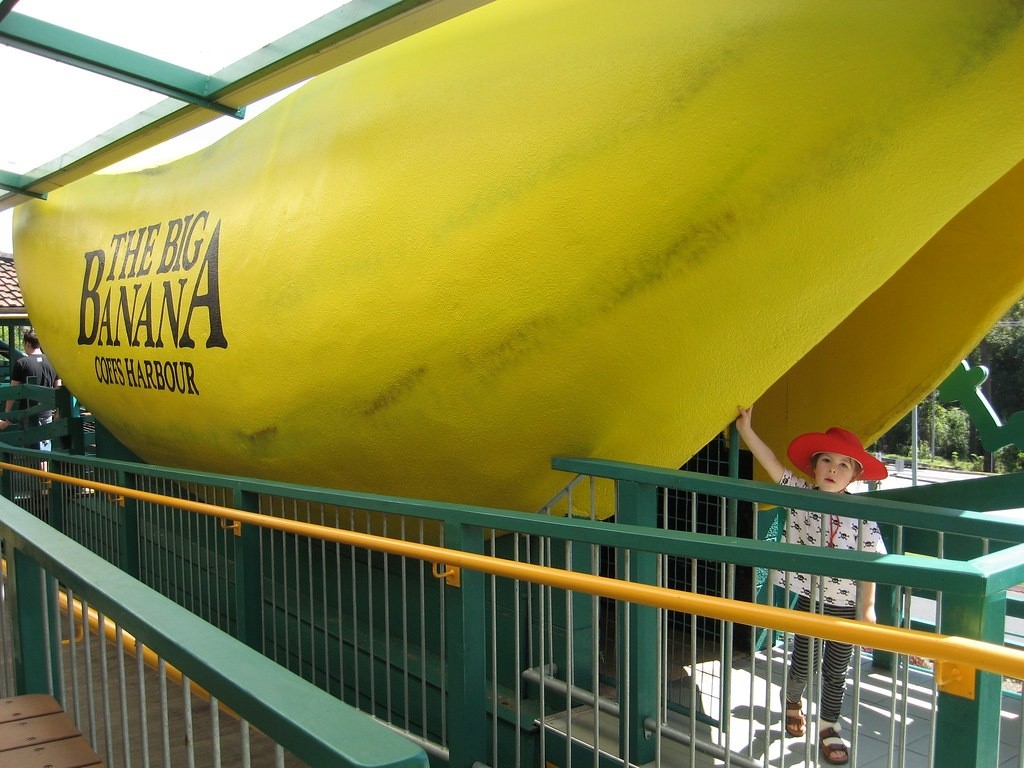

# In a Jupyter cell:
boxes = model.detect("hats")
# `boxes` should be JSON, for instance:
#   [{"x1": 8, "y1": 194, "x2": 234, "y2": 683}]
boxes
[{"x1": 788, "y1": 427, "x2": 888, "y2": 480}]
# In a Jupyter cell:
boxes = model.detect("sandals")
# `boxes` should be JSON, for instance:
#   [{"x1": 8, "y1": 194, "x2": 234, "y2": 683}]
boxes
[
  {"x1": 780, "y1": 688, "x2": 805, "y2": 737},
  {"x1": 819, "y1": 728, "x2": 848, "y2": 764}
]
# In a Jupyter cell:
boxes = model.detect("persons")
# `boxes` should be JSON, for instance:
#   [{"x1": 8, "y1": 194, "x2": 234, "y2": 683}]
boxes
[
  {"x1": 736, "y1": 407, "x2": 888, "y2": 765},
  {"x1": 0, "y1": 332, "x2": 59, "y2": 498}
]
